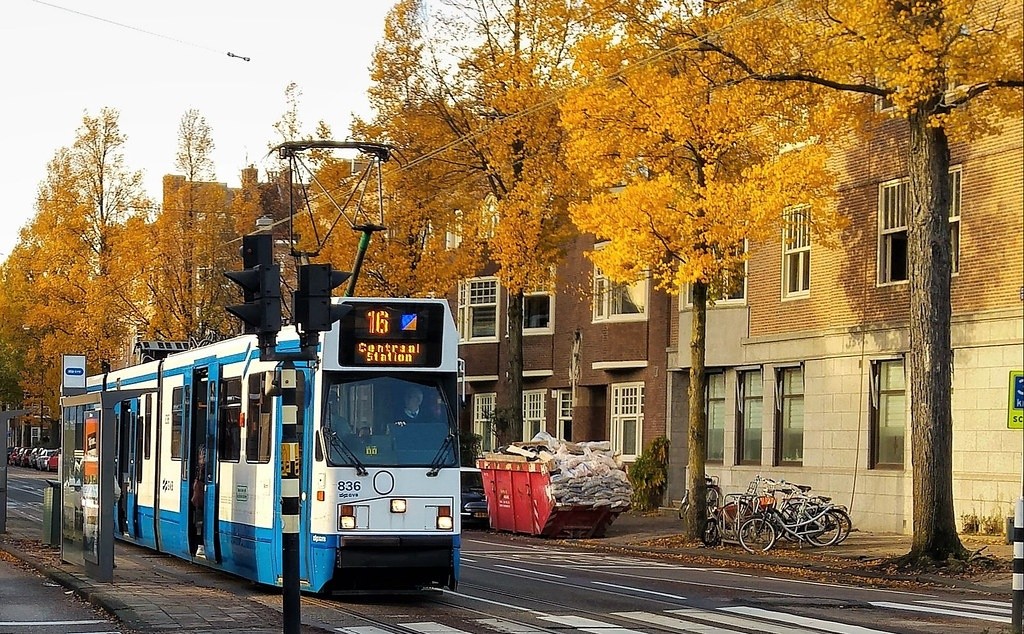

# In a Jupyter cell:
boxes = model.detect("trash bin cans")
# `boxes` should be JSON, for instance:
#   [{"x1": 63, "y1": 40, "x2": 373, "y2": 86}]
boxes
[
  {"x1": 41, "y1": 479, "x2": 61, "y2": 549},
  {"x1": 475, "y1": 440, "x2": 632, "y2": 539}
]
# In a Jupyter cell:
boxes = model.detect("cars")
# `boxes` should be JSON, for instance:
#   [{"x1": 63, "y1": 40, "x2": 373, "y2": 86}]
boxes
[
  {"x1": 37, "y1": 448, "x2": 57, "y2": 471},
  {"x1": 460, "y1": 466, "x2": 489, "y2": 530},
  {"x1": 47, "y1": 448, "x2": 61, "y2": 472},
  {"x1": 6, "y1": 446, "x2": 46, "y2": 469}
]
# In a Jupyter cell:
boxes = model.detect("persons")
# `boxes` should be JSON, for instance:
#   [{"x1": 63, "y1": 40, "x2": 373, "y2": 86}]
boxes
[
  {"x1": 196, "y1": 443, "x2": 207, "y2": 559},
  {"x1": 392, "y1": 386, "x2": 438, "y2": 427}
]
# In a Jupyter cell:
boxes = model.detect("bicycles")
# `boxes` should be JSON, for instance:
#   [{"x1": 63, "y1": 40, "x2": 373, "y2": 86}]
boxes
[
  {"x1": 699, "y1": 474, "x2": 852, "y2": 555},
  {"x1": 679, "y1": 465, "x2": 720, "y2": 520}
]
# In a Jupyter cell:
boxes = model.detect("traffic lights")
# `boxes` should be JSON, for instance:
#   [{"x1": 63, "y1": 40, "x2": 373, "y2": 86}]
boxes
[
  {"x1": 222, "y1": 263, "x2": 282, "y2": 334},
  {"x1": 298, "y1": 263, "x2": 353, "y2": 331}
]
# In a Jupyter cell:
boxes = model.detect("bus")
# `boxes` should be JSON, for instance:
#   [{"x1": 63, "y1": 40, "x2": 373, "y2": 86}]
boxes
[{"x1": 58, "y1": 140, "x2": 468, "y2": 595}]
